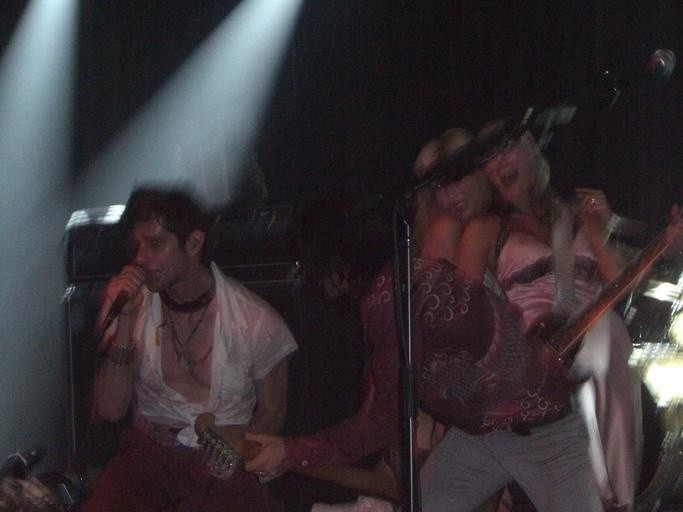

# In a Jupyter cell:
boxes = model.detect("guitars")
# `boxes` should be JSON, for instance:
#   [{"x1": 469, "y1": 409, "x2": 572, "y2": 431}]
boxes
[
  {"x1": 527, "y1": 206, "x2": 682, "y2": 386},
  {"x1": 194, "y1": 413, "x2": 505, "y2": 511}
]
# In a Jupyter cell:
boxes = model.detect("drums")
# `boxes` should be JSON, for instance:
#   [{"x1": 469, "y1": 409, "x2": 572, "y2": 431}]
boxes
[{"x1": 626, "y1": 339, "x2": 683, "y2": 510}]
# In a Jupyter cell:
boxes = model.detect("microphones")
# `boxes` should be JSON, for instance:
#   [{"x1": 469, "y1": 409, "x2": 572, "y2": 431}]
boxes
[
  {"x1": 609, "y1": 48, "x2": 676, "y2": 95},
  {"x1": 97, "y1": 267, "x2": 145, "y2": 336}
]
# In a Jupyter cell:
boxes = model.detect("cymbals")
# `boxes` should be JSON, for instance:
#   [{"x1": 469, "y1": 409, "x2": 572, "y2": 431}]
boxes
[{"x1": 643, "y1": 280, "x2": 682, "y2": 304}]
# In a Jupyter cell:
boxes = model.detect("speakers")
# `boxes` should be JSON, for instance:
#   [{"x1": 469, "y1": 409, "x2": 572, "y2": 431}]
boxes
[{"x1": 67, "y1": 262, "x2": 310, "y2": 457}]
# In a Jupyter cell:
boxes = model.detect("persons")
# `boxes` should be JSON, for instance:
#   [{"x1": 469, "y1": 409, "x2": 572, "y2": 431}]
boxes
[{"x1": 1, "y1": 123, "x2": 682, "y2": 510}]
